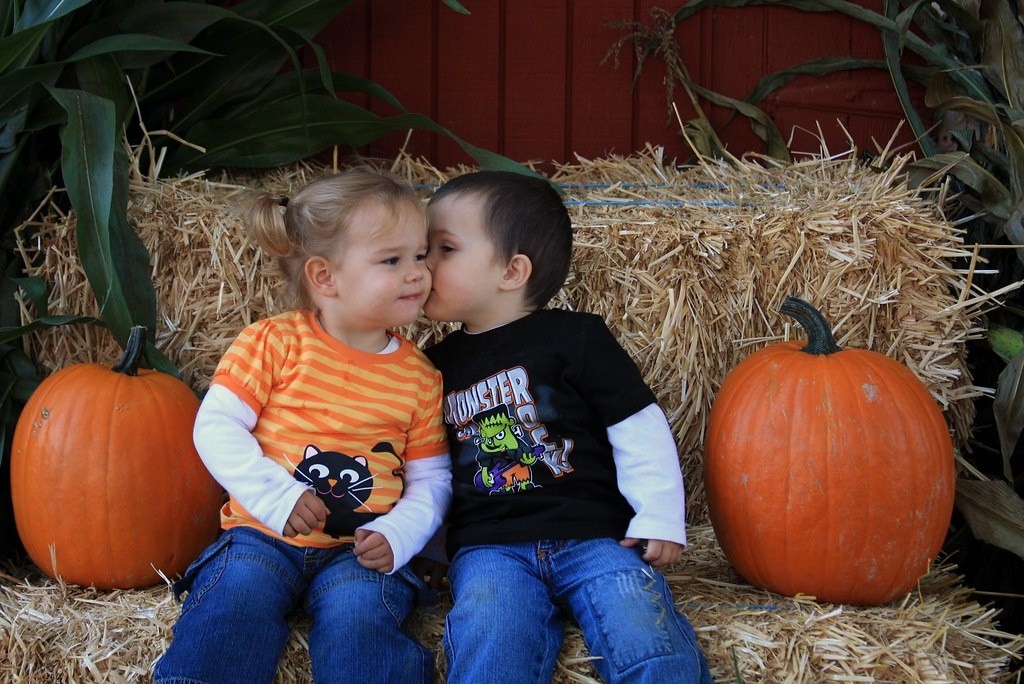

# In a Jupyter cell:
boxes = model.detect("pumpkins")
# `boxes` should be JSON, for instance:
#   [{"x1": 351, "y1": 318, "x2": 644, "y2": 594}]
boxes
[
  {"x1": 704, "y1": 296, "x2": 955, "y2": 608},
  {"x1": 14, "y1": 325, "x2": 221, "y2": 591}
]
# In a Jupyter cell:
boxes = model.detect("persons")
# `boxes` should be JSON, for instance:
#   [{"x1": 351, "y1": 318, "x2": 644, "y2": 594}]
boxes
[
  {"x1": 422, "y1": 171, "x2": 711, "y2": 684},
  {"x1": 148, "y1": 169, "x2": 454, "y2": 684}
]
